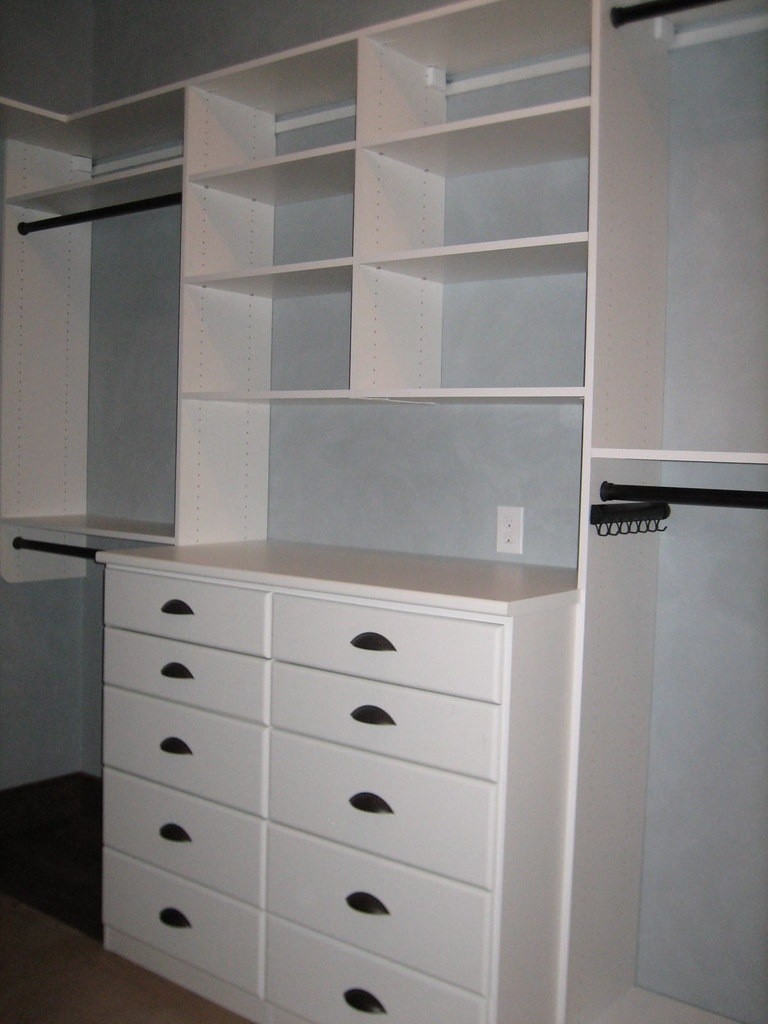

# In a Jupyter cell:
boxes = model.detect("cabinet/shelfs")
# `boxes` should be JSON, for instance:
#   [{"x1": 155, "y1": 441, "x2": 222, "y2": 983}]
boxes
[{"x1": 1, "y1": 0, "x2": 767, "y2": 1022}]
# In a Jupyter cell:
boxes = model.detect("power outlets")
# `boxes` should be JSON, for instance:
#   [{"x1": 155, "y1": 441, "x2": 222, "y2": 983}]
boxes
[{"x1": 497, "y1": 501, "x2": 527, "y2": 556}]
